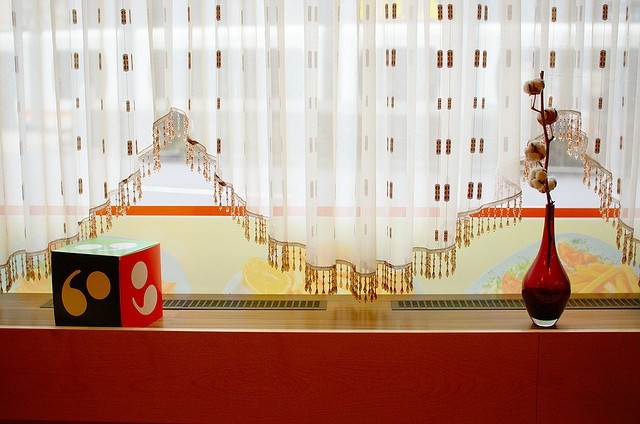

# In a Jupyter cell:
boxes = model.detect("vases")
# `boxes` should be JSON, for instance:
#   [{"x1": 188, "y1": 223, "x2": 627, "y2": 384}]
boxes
[{"x1": 522, "y1": 205, "x2": 571, "y2": 328}]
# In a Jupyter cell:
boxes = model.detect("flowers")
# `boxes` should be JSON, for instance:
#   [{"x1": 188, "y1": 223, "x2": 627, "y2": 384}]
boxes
[{"x1": 523, "y1": 68, "x2": 558, "y2": 271}]
